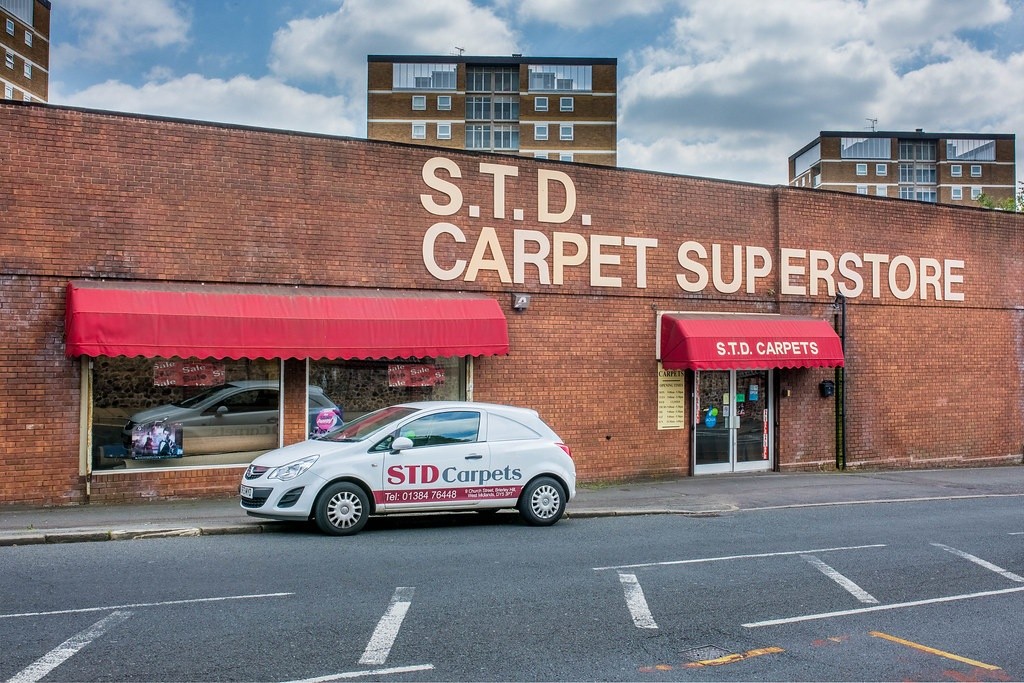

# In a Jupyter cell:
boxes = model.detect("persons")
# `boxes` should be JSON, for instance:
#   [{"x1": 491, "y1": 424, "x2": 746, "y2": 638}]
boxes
[
  {"x1": 143, "y1": 431, "x2": 155, "y2": 450},
  {"x1": 158, "y1": 427, "x2": 177, "y2": 455}
]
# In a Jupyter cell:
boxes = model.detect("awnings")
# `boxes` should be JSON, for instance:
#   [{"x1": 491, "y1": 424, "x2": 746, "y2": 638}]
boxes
[
  {"x1": 661, "y1": 314, "x2": 845, "y2": 371},
  {"x1": 65, "y1": 281, "x2": 510, "y2": 360}
]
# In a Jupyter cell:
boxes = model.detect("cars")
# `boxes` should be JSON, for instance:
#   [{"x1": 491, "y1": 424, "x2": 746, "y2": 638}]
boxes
[
  {"x1": 120, "y1": 379, "x2": 345, "y2": 457},
  {"x1": 237, "y1": 400, "x2": 576, "y2": 537}
]
